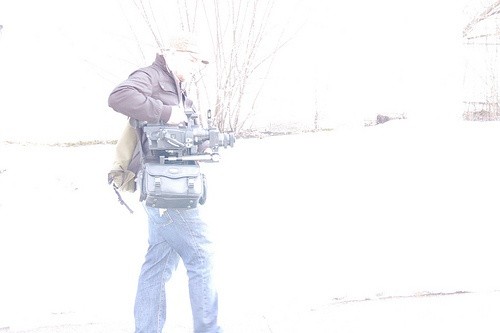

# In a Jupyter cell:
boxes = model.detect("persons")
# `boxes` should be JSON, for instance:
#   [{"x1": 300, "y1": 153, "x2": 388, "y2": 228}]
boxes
[{"x1": 107, "y1": 32, "x2": 229, "y2": 333}]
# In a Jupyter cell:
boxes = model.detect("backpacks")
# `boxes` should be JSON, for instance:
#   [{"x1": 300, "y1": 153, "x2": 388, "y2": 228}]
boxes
[{"x1": 109, "y1": 116, "x2": 143, "y2": 193}]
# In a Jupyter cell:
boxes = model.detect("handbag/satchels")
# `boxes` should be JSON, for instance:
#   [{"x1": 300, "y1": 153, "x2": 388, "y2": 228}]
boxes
[{"x1": 138, "y1": 163, "x2": 207, "y2": 209}]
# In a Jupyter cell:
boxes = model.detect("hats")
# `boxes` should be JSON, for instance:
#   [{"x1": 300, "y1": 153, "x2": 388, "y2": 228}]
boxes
[{"x1": 168, "y1": 33, "x2": 209, "y2": 65}]
